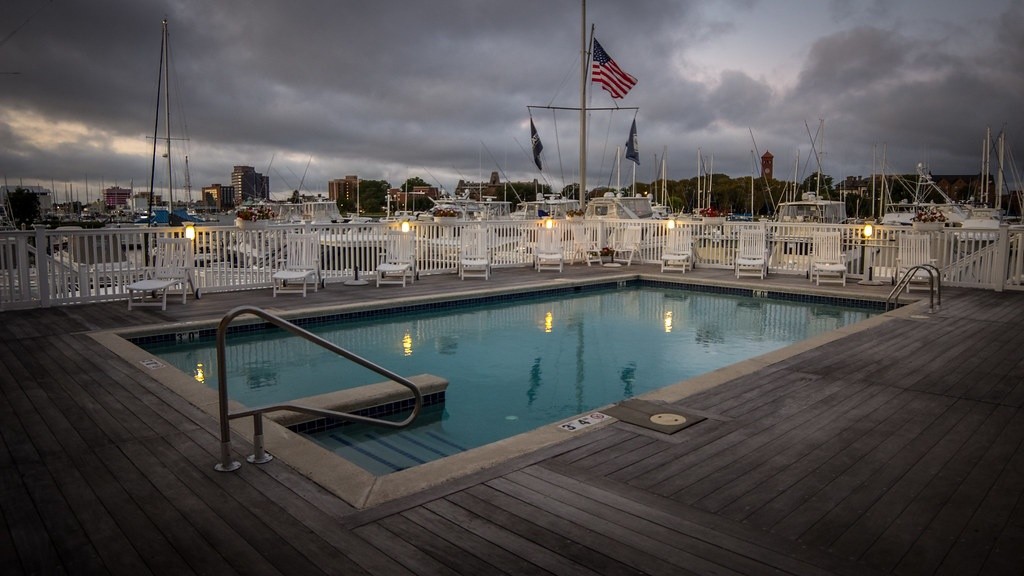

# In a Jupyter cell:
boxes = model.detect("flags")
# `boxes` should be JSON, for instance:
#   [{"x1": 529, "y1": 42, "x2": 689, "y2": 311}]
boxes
[
  {"x1": 591, "y1": 36, "x2": 638, "y2": 100},
  {"x1": 530, "y1": 118, "x2": 544, "y2": 170},
  {"x1": 626, "y1": 118, "x2": 640, "y2": 165}
]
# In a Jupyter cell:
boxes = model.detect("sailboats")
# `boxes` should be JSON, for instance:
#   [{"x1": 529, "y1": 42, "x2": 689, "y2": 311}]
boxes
[{"x1": 1, "y1": 12, "x2": 1022, "y2": 271}]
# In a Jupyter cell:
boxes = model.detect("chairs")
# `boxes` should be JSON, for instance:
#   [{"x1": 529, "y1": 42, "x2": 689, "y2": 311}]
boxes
[
  {"x1": 570, "y1": 224, "x2": 603, "y2": 267},
  {"x1": 735, "y1": 229, "x2": 768, "y2": 280},
  {"x1": 376, "y1": 230, "x2": 415, "y2": 288},
  {"x1": 272, "y1": 233, "x2": 322, "y2": 298},
  {"x1": 613, "y1": 225, "x2": 644, "y2": 267},
  {"x1": 810, "y1": 232, "x2": 847, "y2": 287},
  {"x1": 535, "y1": 226, "x2": 564, "y2": 272},
  {"x1": 126, "y1": 237, "x2": 196, "y2": 311},
  {"x1": 895, "y1": 234, "x2": 939, "y2": 293},
  {"x1": 459, "y1": 228, "x2": 490, "y2": 280},
  {"x1": 661, "y1": 227, "x2": 693, "y2": 273}
]
